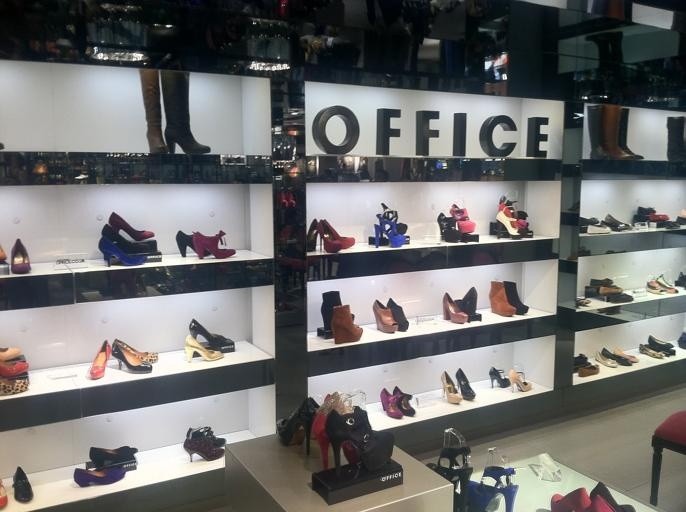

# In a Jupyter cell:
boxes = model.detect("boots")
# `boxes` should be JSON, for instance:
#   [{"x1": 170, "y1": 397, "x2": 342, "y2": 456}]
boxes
[
  {"x1": 321, "y1": 291, "x2": 363, "y2": 344},
  {"x1": 489, "y1": 281, "x2": 529, "y2": 317},
  {"x1": 588, "y1": 105, "x2": 643, "y2": 160},
  {"x1": 139, "y1": 69, "x2": 210, "y2": 154}
]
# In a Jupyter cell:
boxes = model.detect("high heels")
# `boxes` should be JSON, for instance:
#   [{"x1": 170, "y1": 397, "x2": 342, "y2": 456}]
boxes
[
  {"x1": 74, "y1": 446, "x2": 137, "y2": 487},
  {"x1": 185, "y1": 320, "x2": 232, "y2": 362},
  {"x1": 90, "y1": 340, "x2": 157, "y2": 379},
  {"x1": 184, "y1": 426, "x2": 225, "y2": 461},
  {"x1": 276, "y1": 397, "x2": 393, "y2": 475},
  {"x1": 438, "y1": 204, "x2": 475, "y2": 242},
  {"x1": 489, "y1": 367, "x2": 531, "y2": 393},
  {"x1": 443, "y1": 287, "x2": 477, "y2": 323},
  {"x1": 426, "y1": 447, "x2": 518, "y2": 512},
  {"x1": 176, "y1": 231, "x2": 235, "y2": 258},
  {"x1": 381, "y1": 386, "x2": 415, "y2": 418},
  {"x1": 375, "y1": 203, "x2": 407, "y2": 248},
  {"x1": 442, "y1": 368, "x2": 475, "y2": 403},
  {"x1": 374, "y1": 298, "x2": 409, "y2": 333},
  {"x1": 99, "y1": 212, "x2": 154, "y2": 267},
  {"x1": 307, "y1": 219, "x2": 355, "y2": 253},
  {"x1": 11, "y1": 239, "x2": 30, "y2": 273},
  {"x1": 496, "y1": 202, "x2": 533, "y2": 239}
]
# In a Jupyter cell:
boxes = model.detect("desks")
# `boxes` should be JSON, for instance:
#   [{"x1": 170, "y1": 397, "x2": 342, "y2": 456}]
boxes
[{"x1": 222, "y1": 431, "x2": 456, "y2": 511}]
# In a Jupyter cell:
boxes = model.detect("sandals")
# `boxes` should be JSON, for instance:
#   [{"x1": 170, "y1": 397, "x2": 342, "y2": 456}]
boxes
[{"x1": 648, "y1": 272, "x2": 686, "y2": 294}]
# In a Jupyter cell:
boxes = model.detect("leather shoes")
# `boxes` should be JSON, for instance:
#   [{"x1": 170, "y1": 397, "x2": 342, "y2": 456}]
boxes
[
  {"x1": 590, "y1": 279, "x2": 622, "y2": 294},
  {"x1": 0, "y1": 466, "x2": 32, "y2": 507},
  {"x1": 579, "y1": 214, "x2": 629, "y2": 233},
  {"x1": 574, "y1": 348, "x2": 638, "y2": 377},
  {"x1": 551, "y1": 482, "x2": 635, "y2": 512},
  {"x1": 0, "y1": 348, "x2": 28, "y2": 377},
  {"x1": 640, "y1": 336, "x2": 675, "y2": 358},
  {"x1": 634, "y1": 207, "x2": 686, "y2": 229}
]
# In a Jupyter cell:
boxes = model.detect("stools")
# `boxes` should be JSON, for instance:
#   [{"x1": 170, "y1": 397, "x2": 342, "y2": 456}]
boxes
[{"x1": 648, "y1": 412, "x2": 685, "y2": 506}]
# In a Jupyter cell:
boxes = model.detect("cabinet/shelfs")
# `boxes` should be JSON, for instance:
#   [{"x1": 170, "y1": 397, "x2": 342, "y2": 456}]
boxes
[
  {"x1": 1, "y1": 0, "x2": 307, "y2": 511},
  {"x1": 270, "y1": 2, "x2": 582, "y2": 459},
  {"x1": 557, "y1": 0, "x2": 686, "y2": 417}
]
